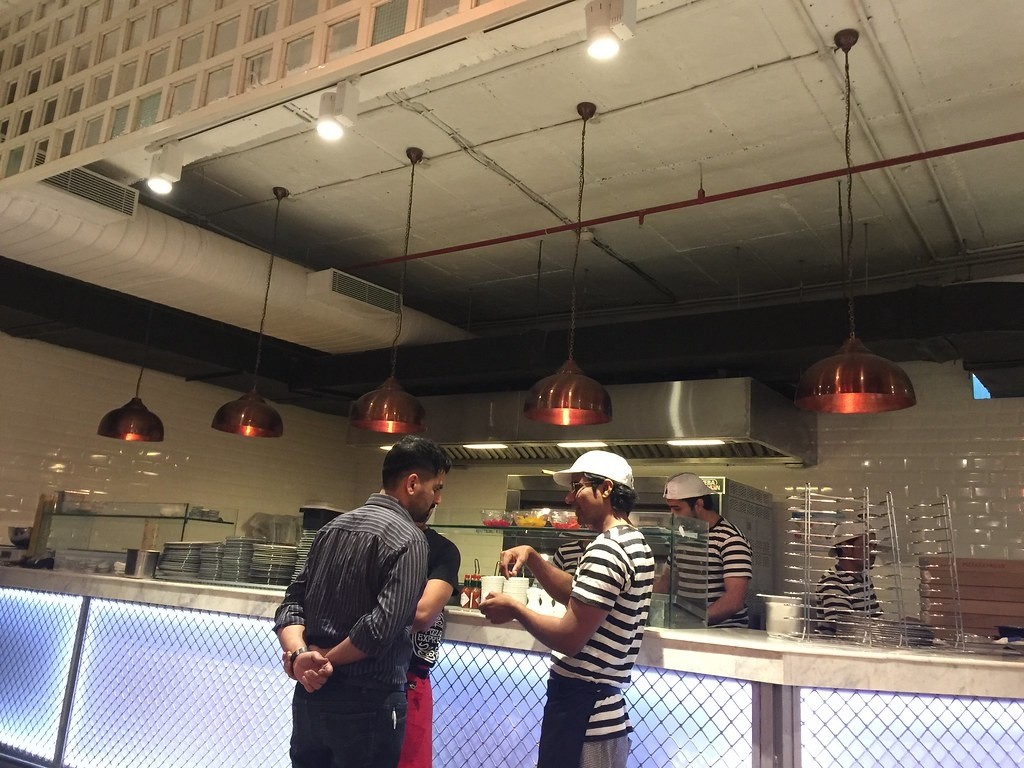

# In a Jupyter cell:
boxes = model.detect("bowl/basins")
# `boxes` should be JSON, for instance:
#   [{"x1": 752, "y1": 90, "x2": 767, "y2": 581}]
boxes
[
  {"x1": 480, "y1": 511, "x2": 513, "y2": 526},
  {"x1": 512, "y1": 510, "x2": 548, "y2": 527},
  {"x1": 7, "y1": 525, "x2": 32, "y2": 548},
  {"x1": 549, "y1": 508, "x2": 581, "y2": 528},
  {"x1": 994, "y1": 625, "x2": 1024, "y2": 639}
]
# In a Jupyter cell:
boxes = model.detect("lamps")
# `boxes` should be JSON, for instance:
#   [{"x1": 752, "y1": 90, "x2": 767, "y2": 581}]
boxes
[
  {"x1": 350, "y1": 147, "x2": 425, "y2": 435},
  {"x1": 796, "y1": 29, "x2": 916, "y2": 413},
  {"x1": 212, "y1": 186, "x2": 288, "y2": 438},
  {"x1": 318, "y1": 80, "x2": 352, "y2": 141},
  {"x1": 585, "y1": 0, "x2": 634, "y2": 61},
  {"x1": 98, "y1": 303, "x2": 165, "y2": 441},
  {"x1": 524, "y1": 102, "x2": 612, "y2": 425},
  {"x1": 145, "y1": 139, "x2": 182, "y2": 195}
]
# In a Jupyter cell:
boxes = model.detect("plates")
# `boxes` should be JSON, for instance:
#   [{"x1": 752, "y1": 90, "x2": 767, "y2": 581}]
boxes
[
  {"x1": 157, "y1": 530, "x2": 319, "y2": 586},
  {"x1": 481, "y1": 575, "x2": 529, "y2": 607}
]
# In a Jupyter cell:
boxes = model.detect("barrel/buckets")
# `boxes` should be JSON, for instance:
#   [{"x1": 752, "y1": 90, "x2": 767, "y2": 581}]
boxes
[{"x1": 124, "y1": 548, "x2": 160, "y2": 580}]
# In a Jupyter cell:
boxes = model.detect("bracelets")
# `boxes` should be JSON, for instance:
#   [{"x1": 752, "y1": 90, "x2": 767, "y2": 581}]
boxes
[{"x1": 290, "y1": 647, "x2": 310, "y2": 666}]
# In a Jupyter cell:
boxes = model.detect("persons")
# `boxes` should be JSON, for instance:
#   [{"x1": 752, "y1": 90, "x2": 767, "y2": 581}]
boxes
[
  {"x1": 398, "y1": 505, "x2": 460, "y2": 768},
  {"x1": 273, "y1": 434, "x2": 453, "y2": 768},
  {"x1": 815, "y1": 522, "x2": 884, "y2": 639},
  {"x1": 478, "y1": 450, "x2": 656, "y2": 768},
  {"x1": 652, "y1": 473, "x2": 752, "y2": 628},
  {"x1": 553, "y1": 524, "x2": 596, "y2": 574}
]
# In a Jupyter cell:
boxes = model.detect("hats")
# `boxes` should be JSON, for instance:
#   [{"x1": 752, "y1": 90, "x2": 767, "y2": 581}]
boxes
[
  {"x1": 828, "y1": 519, "x2": 877, "y2": 557},
  {"x1": 663, "y1": 472, "x2": 717, "y2": 500},
  {"x1": 553, "y1": 450, "x2": 635, "y2": 491}
]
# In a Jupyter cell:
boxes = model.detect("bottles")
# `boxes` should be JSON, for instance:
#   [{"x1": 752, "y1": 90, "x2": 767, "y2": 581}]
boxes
[
  {"x1": 525, "y1": 587, "x2": 567, "y2": 618},
  {"x1": 459, "y1": 574, "x2": 481, "y2": 608}
]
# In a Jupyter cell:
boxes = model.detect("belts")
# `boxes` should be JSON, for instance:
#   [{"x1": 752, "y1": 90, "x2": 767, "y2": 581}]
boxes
[{"x1": 331, "y1": 670, "x2": 411, "y2": 693}]
